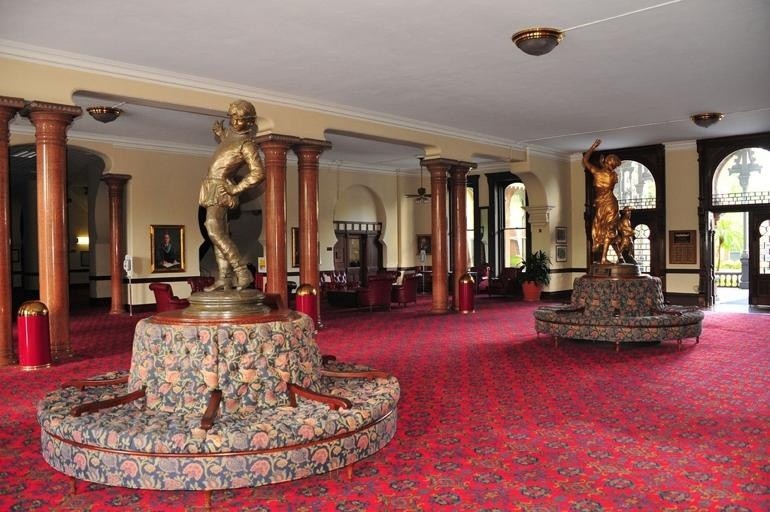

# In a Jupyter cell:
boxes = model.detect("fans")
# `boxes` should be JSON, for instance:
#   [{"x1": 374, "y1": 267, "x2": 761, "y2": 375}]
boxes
[{"x1": 402, "y1": 158, "x2": 432, "y2": 204}]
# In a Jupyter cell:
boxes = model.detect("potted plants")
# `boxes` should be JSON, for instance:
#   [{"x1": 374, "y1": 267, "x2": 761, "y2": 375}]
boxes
[{"x1": 514, "y1": 249, "x2": 553, "y2": 301}]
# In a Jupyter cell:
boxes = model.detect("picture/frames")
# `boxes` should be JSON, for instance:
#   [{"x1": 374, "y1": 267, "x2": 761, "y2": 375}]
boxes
[
  {"x1": 416, "y1": 234, "x2": 432, "y2": 255},
  {"x1": 149, "y1": 224, "x2": 186, "y2": 273},
  {"x1": 554, "y1": 226, "x2": 568, "y2": 262}
]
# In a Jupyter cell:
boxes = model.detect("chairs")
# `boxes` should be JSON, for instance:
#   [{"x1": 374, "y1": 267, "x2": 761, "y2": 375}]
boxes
[
  {"x1": 356, "y1": 270, "x2": 423, "y2": 310},
  {"x1": 36, "y1": 308, "x2": 400, "y2": 491},
  {"x1": 532, "y1": 275, "x2": 704, "y2": 354},
  {"x1": 149, "y1": 276, "x2": 215, "y2": 313},
  {"x1": 470, "y1": 264, "x2": 518, "y2": 300}
]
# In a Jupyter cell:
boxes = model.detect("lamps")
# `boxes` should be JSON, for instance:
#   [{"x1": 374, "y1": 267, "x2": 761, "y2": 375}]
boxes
[
  {"x1": 512, "y1": 27, "x2": 564, "y2": 57},
  {"x1": 87, "y1": 107, "x2": 123, "y2": 124},
  {"x1": 690, "y1": 112, "x2": 723, "y2": 128}
]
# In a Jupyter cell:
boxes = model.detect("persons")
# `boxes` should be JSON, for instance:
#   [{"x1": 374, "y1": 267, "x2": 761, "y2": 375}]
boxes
[
  {"x1": 581, "y1": 137, "x2": 624, "y2": 265},
  {"x1": 155, "y1": 230, "x2": 180, "y2": 269},
  {"x1": 618, "y1": 206, "x2": 634, "y2": 263},
  {"x1": 419, "y1": 237, "x2": 432, "y2": 254},
  {"x1": 200, "y1": 99, "x2": 266, "y2": 293}
]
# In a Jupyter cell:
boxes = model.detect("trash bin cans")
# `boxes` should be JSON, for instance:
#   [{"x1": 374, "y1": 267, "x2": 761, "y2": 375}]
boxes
[
  {"x1": 459, "y1": 274, "x2": 475, "y2": 314},
  {"x1": 17, "y1": 303, "x2": 51, "y2": 370},
  {"x1": 294, "y1": 285, "x2": 319, "y2": 331}
]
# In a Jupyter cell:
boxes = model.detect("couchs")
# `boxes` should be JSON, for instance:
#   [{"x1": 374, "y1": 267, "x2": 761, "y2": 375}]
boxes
[{"x1": 319, "y1": 270, "x2": 360, "y2": 300}]
